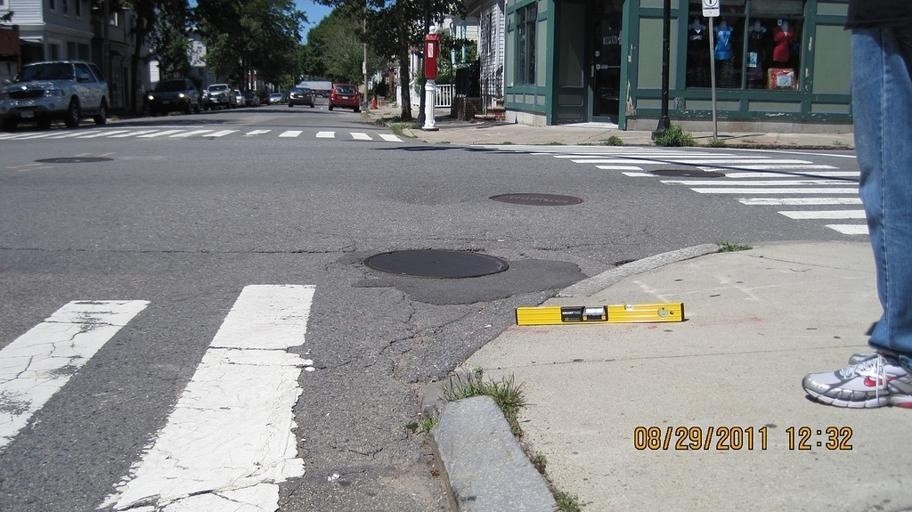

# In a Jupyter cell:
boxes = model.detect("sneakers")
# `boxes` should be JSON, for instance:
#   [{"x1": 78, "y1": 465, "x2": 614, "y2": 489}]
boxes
[{"x1": 802, "y1": 352, "x2": 912, "y2": 410}]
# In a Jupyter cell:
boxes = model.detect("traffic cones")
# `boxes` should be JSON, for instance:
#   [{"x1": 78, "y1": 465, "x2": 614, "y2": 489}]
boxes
[{"x1": 371, "y1": 96, "x2": 376, "y2": 109}]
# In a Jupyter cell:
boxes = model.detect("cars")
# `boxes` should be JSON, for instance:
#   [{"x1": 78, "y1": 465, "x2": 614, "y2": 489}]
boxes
[
  {"x1": 288, "y1": 88, "x2": 315, "y2": 107},
  {"x1": 201, "y1": 83, "x2": 285, "y2": 111},
  {"x1": 329, "y1": 84, "x2": 360, "y2": 112}
]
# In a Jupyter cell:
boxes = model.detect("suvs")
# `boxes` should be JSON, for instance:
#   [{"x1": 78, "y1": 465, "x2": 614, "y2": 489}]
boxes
[
  {"x1": 0, "y1": 61, "x2": 111, "y2": 129},
  {"x1": 147, "y1": 78, "x2": 200, "y2": 116}
]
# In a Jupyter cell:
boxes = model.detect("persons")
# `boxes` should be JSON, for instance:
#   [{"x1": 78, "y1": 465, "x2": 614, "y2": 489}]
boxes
[
  {"x1": 802, "y1": 0, "x2": 912, "y2": 408},
  {"x1": 688, "y1": 19, "x2": 710, "y2": 63},
  {"x1": 773, "y1": 22, "x2": 794, "y2": 63},
  {"x1": 713, "y1": 21, "x2": 734, "y2": 61},
  {"x1": 749, "y1": 22, "x2": 767, "y2": 66}
]
